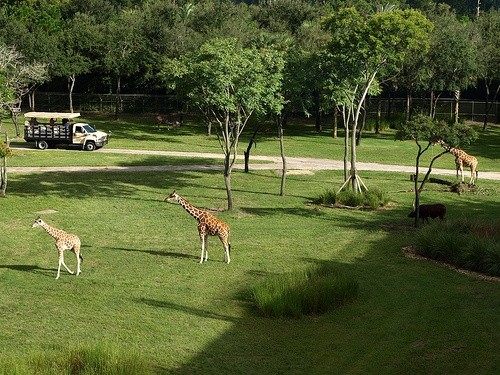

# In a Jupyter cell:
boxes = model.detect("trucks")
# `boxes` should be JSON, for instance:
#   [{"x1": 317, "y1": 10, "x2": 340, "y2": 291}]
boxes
[{"x1": 24, "y1": 112, "x2": 108, "y2": 151}]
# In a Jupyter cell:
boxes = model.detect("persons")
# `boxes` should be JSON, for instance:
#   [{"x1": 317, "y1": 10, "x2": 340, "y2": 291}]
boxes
[
  {"x1": 50, "y1": 118, "x2": 57, "y2": 126},
  {"x1": 62, "y1": 118, "x2": 69, "y2": 126},
  {"x1": 30, "y1": 117, "x2": 38, "y2": 125}
]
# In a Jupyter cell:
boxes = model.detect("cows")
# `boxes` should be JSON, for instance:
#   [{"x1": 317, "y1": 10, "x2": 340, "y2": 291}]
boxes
[{"x1": 407, "y1": 199, "x2": 447, "y2": 225}]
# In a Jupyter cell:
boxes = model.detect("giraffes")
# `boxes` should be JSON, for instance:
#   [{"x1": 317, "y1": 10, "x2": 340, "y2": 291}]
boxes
[
  {"x1": 29, "y1": 216, "x2": 84, "y2": 281},
  {"x1": 432, "y1": 138, "x2": 479, "y2": 185},
  {"x1": 163, "y1": 189, "x2": 232, "y2": 265}
]
